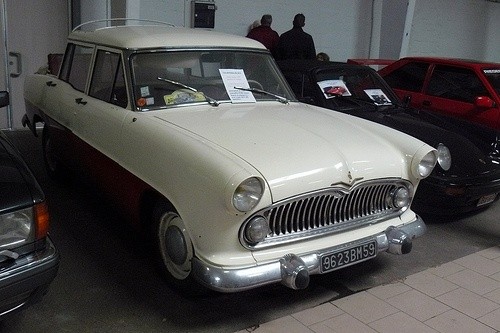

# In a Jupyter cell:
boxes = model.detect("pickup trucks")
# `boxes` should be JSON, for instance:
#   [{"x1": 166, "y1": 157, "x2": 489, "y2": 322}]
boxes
[{"x1": 22, "y1": 18, "x2": 441, "y2": 292}]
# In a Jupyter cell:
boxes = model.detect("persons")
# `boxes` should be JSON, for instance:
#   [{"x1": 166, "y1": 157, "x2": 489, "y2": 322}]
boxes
[
  {"x1": 280, "y1": 14, "x2": 316, "y2": 61},
  {"x1": 317, "y1": 52, "x2": 330, "y2": 62},
  {"x1": 246, "y1": 14, "x2": 284, "y2": 60}
]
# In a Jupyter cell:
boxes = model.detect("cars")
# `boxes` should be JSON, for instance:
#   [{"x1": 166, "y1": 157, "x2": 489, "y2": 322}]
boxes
[
  {"x1": 0, "y1": 90, "x2": 60, "y2": 319},
  {"x1": 347, "y1": 57, "x2": 499, "y2": 134},
  {"x1": 273, "y1": 59, "x2": 500, "y2": 222}
]
